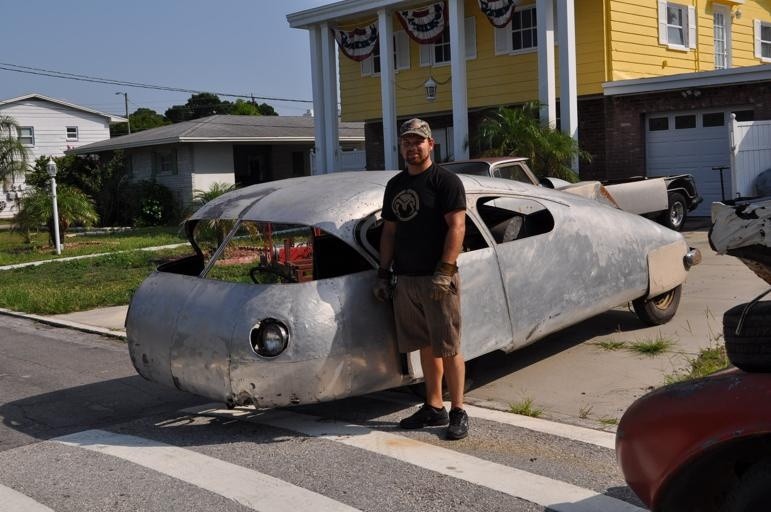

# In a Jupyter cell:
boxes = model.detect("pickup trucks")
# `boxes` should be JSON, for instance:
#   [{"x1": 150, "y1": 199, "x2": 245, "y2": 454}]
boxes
[{"x1": 439, "y1": 157, "x2": 704, "y2": 232}]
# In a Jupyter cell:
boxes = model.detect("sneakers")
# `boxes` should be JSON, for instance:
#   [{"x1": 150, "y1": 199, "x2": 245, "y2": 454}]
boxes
[
  {"x1": 400, "y1": 405, "x2": 449, "y2": 430},
  {"x1": 445, "y1": 407, "x2": 469, "y2": 439}
]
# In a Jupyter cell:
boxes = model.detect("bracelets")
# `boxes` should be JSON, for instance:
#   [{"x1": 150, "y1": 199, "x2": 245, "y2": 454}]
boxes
[
  {"x1": 377, "y1": 268, "x2": 392, "y2": 278},
  {"x1": 438, "y1": 261, "x2": 459, "y2": 276}
]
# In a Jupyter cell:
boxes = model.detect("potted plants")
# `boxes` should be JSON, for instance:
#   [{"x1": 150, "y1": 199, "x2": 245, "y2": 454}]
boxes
[{"x1": 8, "y1": 179, "x2": 101, "y2": 244}]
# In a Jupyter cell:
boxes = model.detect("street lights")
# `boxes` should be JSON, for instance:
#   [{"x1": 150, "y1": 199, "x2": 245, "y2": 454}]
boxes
[
  {"x1": 115, "y1": 92, "x2": 131, "y2": 135},
  {"x1": 46, "y1": 156, "x2": 61, "y2": 255}
]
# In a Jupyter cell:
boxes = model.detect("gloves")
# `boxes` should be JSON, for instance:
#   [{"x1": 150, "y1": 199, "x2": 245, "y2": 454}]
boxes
[
  {"x1": 429, "y1": 261, "x2": 457, "y2": 300},
  {"x1": 372, "y1": 268, "x2": 392, "y2": 302}
]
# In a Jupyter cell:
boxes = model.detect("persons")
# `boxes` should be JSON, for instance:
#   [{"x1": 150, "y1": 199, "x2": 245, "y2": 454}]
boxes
[{"x1": 372, "y1": 117, "x2": 470, "y2": 440}]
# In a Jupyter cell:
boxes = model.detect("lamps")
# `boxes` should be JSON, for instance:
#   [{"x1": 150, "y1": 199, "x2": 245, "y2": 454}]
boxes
[
  {"x1": 425, "y1": 44, "x2": 437, "y2": 102},
  {"x1": 735, "y1": 10, "x2": 742, "y2": 19}
]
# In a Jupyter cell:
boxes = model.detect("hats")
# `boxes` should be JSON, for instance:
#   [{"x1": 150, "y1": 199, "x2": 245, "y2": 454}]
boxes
[{"x1": 400, "y1": 118, "x2": 432, "y2": 139}]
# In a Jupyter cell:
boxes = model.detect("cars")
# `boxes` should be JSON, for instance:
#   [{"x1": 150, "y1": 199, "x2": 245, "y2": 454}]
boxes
[
  {"x1": 614, "y1": 198, "x2": 770, "y2": 512},
  {"x1": 125, "y1": 169, "x2": 702, "y2": 410}
]
